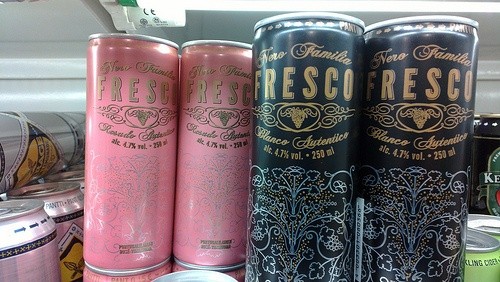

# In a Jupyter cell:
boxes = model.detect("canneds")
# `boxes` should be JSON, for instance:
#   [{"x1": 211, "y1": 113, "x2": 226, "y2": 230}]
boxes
[
  {"x1": 0, "y1": 111, "x2": 85, "y2": 282},
  {"x1": 171, "y1": 39, "x2": 255, "y2": 271},
  {"x1": 83, "y1": 32, "x2": 178, "y2": 273},
  {"x1": 245, "y1": 11, "x2": 365, "y2": 282},
  {"x1": 82, "y1": 260, "x2": 246, "y2": 282},
  {"x1": 462, "y1": 113, "x2": 500, "y2": 282},
  {"x1": 349, "y1": 15, "x2": 480, "y2": 282}
]
